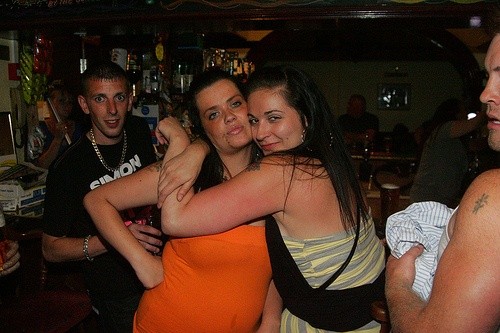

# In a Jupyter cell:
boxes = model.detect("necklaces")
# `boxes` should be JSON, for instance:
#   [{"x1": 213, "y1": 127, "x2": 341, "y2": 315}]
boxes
[
  {"x1": 89, "y1": 127, "x2": 127, "y2": 173},
  {"x1": 222, "y1": 161, "x2": 232, "y2": 180}
]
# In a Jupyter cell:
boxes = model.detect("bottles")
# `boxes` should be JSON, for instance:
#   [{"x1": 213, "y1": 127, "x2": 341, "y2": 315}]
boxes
[
  {"x1": 175, "y1": 61, "x2": 194, "y2": 94},
  {"x1": 126, "y1": 52, "x2": 161, "y2": 106},
  {"x1": 358, "y1": 149, "x2": 376, "y2": 182},
  {"x1": 208, "y1": 50, "x2": 256, "y2": 84}
]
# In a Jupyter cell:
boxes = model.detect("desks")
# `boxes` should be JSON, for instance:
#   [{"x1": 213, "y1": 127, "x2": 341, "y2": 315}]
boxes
[
  {"x1": 0, "y1": 217, "x2": 100, "y2": 333},
  {"x1": 350, "y1": 155, "x2": 415, "y2": 199}
]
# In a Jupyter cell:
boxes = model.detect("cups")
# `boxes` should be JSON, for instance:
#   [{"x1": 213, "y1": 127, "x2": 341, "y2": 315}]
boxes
[
  {"x1": 146, "y1": 219, "x2": 169, "y2": 257},
  {"x1": 380, "y1": 184, "x2": 401, "y2": 225},
  {"x1": 0, "y1": 240, "x2": 10, "y2": 272}
]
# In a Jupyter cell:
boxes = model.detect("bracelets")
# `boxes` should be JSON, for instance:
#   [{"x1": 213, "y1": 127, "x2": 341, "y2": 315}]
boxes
[{"x1": 84, "y1": 234, "x2": 94, "y2": 262}]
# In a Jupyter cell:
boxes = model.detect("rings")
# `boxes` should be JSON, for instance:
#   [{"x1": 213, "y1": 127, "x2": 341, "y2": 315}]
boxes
[{"x1": 65, "y1": 126, "x2": 67, "y2": 129}]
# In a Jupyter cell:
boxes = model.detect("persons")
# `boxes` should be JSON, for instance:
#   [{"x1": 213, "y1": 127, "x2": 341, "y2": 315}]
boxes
[{"x1": 0, "y1": 32, "x2": 500, "y2": 333}]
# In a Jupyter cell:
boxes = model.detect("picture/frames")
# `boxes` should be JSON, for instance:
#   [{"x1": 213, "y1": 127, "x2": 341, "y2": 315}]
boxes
[{"x1": 376, "y1": 83, "x2": 412, "y2": 110}]
